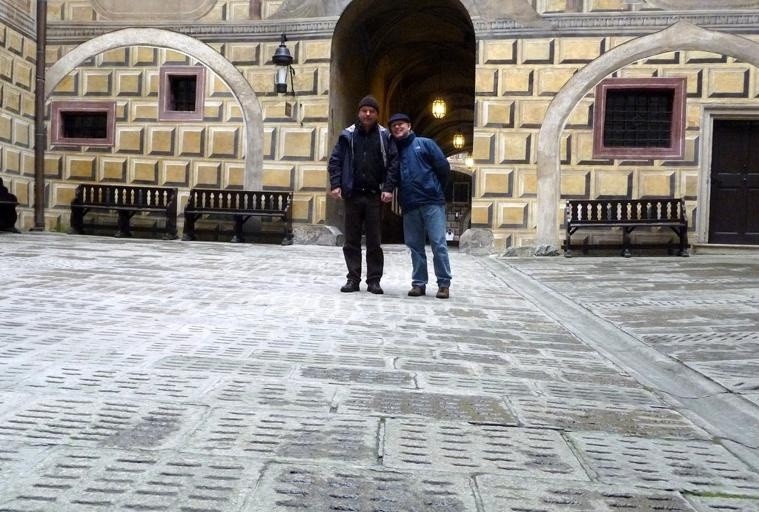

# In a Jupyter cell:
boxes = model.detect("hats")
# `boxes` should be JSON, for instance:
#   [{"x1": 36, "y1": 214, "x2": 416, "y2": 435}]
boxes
[
  {"x1": 358, "y1": 94, "x2": 381, "y2": 114},
  {"x1": 387, "y1": 112, "x2": 412, "y2": 126}
]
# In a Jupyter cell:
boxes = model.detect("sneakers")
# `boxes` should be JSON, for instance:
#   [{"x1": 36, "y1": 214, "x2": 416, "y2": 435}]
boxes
[
  {"x1": 407, "y1": 284, "x2": 427, "y2": 297},
  {"x1": 340, "y1": 278, "x2": 362, "y2": 293},
  {"x1": 436, "y1": 286, "x2": 450, "y2": 299},
  {"x1": 366, "y1": 280, "x2": 385, "y2": 294}
]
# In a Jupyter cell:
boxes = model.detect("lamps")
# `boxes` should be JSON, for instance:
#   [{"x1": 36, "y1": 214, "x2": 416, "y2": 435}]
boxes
[
  {"x1": 272, "y1": 32, "x2": 295, "y2": 93},
  {"x1": 431, "y1": 67, "x2": 448, "y2": 118},
  {"x1": 453, "y1": 96, "x2": 466, "y2": 150},
  {"x1": 465, "y1": 151, "x2": 473, "y2": 170}
]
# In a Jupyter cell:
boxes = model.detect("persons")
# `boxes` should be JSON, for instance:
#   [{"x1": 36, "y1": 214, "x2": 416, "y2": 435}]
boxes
[
  {"x1": 383, "y1": 110, "x2": 455, "y2": 300},
  {"x1": 325, "y1": 95, "x2": 398, "y2": 293}
]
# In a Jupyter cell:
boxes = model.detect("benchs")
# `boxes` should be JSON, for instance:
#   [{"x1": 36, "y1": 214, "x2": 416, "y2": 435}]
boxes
[
  {"x1": 560, "y1": 198, "x2": 691, "y2": 257},
  {"x1": 181, "y1": 189, "x2": 294, "y2": 245},
  {"x1": 70, "y1": 183, "x2": 177, "y2": 240}
]
